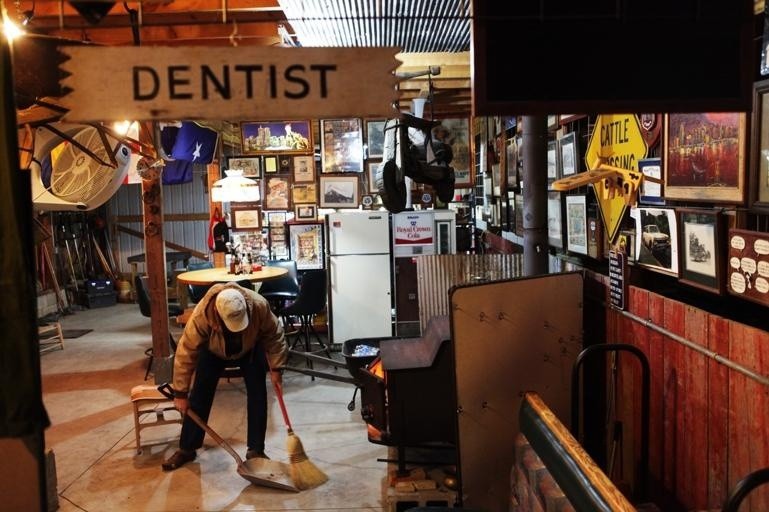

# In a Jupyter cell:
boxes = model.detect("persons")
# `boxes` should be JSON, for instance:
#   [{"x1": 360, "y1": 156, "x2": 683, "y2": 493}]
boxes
[{"x1": 161, "y1": 283, "x2": 286, "y2": 471}]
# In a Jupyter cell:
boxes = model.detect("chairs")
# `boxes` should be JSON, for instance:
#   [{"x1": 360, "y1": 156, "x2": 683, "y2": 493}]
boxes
[
  {"x1": 186, "y1": 262, "x2": 213, "y2": 302},
  {"x1": 281, "y1": 270, "x2": 339, "y2": 381},
  {"x1": 259, "y1": 261, "x2": 305, "y2": 353},
  {"x1": 133, "y1": 271, "x2": 183, "y2": 381}
]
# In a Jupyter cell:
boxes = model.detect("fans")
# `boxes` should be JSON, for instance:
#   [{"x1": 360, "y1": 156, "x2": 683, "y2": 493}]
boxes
[{"x1": 30, "y1": 123, "x2": 131, "y2": 212}]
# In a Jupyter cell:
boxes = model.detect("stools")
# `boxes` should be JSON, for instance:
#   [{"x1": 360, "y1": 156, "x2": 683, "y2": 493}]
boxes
[{"x1": 130, "y1": 384, "x2": 184, "y2": 454}]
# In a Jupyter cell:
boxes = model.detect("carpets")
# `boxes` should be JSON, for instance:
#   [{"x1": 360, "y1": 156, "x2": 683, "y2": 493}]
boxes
[{"x1": 39, "y1": 328, "x2": 93, "y2": 338}]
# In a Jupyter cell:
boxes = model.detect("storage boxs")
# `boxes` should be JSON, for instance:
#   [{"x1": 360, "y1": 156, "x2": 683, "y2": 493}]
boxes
[
  {"x1": 84, "y1": 280, "x2": 114, "y2": 294},
  {"x1": 80, "y1": 294, "x2": 116, "y2": 310}
]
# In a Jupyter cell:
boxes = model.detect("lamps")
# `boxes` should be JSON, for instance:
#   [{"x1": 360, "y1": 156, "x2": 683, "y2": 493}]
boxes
[{"x1": 209, "y1": 124, "x2": 261, "y2": 202}]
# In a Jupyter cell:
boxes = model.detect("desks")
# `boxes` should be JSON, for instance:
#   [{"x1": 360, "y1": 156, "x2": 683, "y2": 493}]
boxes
[
  {"x1": 177, "y1": 266, "x2": 288, "y2": 307},
  {"x1": 127, "y1": 250, "x2": 193, "y2": 305}
]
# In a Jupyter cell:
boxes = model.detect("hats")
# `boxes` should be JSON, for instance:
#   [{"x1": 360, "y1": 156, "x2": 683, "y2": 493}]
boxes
[{"x1": 213, "y1": 289, "x2": 251, "y2": 334}]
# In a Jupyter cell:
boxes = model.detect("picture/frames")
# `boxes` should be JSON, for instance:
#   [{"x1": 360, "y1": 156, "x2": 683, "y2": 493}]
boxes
[
  {"x1": 480, "y1": 116, "x2": 489, "y2": 173},
  {"x1": 637, "y1": 158, "x2": 665, "y2": 206},
  {"x1": 367, "y1": 119, "x2": 386, "y2": 159},
  {"x1": 447, "y1": 201, "x2": 475, "y2": 225},
  {"x1": 505, "y1": 115, "x2": 517, "y2": 132},
  {"x1": 548, "y1": 114, "x2": 559, "y2": 132},
  {"x1": 492, "y1": 164, "x2": 501, "y2": 198},
  {"x1": 367, "y1": 160, "x2": 382, "y2": 194},
  {"x1": 441, "y1": 118, "x2": 473, "y2": 189},
  {"x1": 241, "y1": 117, "x2": 314, "y2": 155},
  {"x1": 362, "y1": 195, "x2": 374, "y2": 210},
  {"x1": 495, "y1": 116, "x2": 503, "y2": 136},
  {"x1": 505, "y1": 133, "x2": 520, "y2": 190},
  {"x1": 228, "y1": 156, "x2": 261, "y2": 179},
  {"x1": 287, "y1": 222, "x2": 326, "y2": 272},
  {"x1": 483, "y1": 199, "x2": 501, "y2": 230},
  {"x1": 748, "y1": 79, "x2": 769, "y2": 215},
  {"x1": 318, "y1": 175, "x2": 360, "y2": 210},
  {"x1": 558, "y1": 113, "x2": 585, "y2": 126},
  {"x1": 293, "y1": 203, "x2": 319, "y2": 223},
  {"x1": 558, "y1": 131, "x2": 580, "y2": 178},
  {"x1": 292, "y1": 153, "x2": 315, "y2": 184},
  {"x1": 507, "y1": 191, "x2": 515, "y2": 232},
  {"x1": 548, "y1": 138, "x2": 559, "y2": 183},
  {"x1": 565, "y1": 194, "x2": 590, "y2": 257},
  {"x1": 675, "y1": 208, "x2": 723, "y2": 297},
  {"x1": 636, "y1": 207, "x2": 679, "y2": 280},
  {"x1": 500, "y1": 196, "x2": 506, "y2": 230},
  {"x1": 319, "y1": 116, "x2": 365, "y2": 175},
  {"x1": 515, "y1": 194, "x2": 525, "y2": 237},
  {"x1": 605, "y1": 216, "x2": 636, "y2": 267},
  {"x1": 548, "y1": 190, "x2": 565, "y2": 251},
  {"x1": 265, "y1": 155, "x2": 277, "y2": 174},
  {"x1": 230, "y1": 206, "x2": 262, "y2": 233},
  {"x1": 661, "y1": 114, "x2": 749, "y2": 207},
  {"x1": 291, "y1": 184, "x2": 318, "y2": 204}
]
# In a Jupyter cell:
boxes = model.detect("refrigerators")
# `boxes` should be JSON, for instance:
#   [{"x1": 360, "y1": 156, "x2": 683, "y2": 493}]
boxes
[{"x1": 327, "y1": 210, "x2": 394, "y2": 345}]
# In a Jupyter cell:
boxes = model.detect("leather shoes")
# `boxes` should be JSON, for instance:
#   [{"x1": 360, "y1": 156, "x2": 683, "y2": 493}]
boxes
[
  {"x1": 245, "y1": 449, "x2": 270, "y2": 462},
  {"x1": 162, "y1": 450, "x2": 199, "y2": 471}
]
